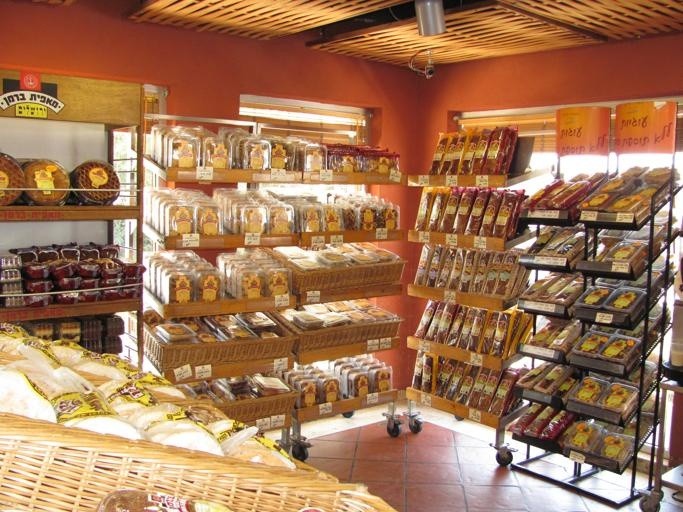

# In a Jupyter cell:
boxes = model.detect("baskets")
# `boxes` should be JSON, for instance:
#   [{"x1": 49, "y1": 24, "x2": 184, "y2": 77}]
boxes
[
  {"x1": 144, "y1": 300, "x2": 404, "y2": 374},
  {"x1": 272, "y1": 247, "x2": 407, "y2": 293},
  {"x1": 213, "y1": 375, "x2": 299, "y2": 421},
  {"x1": 0, "y1": 351, "x2": 402, "y2": 511}
]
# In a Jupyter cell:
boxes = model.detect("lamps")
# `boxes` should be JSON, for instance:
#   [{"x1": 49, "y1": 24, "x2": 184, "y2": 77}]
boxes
[{"x1": 414, "y1": 0, "x2": 448, "y2": 38}]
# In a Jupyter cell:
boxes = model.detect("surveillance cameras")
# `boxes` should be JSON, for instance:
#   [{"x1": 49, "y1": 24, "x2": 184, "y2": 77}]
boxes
[{"x1": 425, "y1": 65, "x2": 433, "y2": 79}]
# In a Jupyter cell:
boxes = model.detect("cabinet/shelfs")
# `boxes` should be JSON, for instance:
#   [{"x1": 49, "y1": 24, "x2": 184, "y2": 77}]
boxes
[{"x1": 2, "y1": 69, "x2": 149, "y2": 369}]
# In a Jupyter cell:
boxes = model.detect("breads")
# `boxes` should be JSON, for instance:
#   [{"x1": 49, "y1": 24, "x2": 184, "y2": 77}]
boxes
[
  {"x1": 412, "y1": 126, "x2": 681, "y2": 472},
  {"x1": 0, "y1": 120, "x2": 406, "y2": 512}
]
